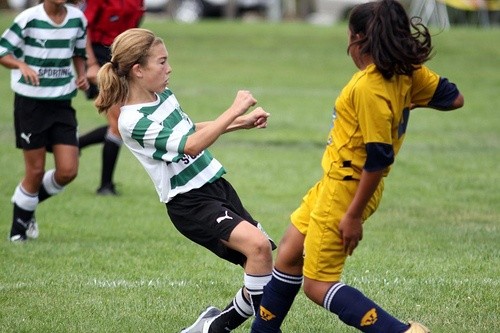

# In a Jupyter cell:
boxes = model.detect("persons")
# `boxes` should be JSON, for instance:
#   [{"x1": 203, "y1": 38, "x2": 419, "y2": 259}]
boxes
[
  {"x1": 0, "y1": 0, "x2": 145, "y2": 243},
  {"x1": 250, "y1": 0, "x2": 464, "y2": 333},
  {"x1": 94, "y1": 28, "x2": 277, "y2": 333}
]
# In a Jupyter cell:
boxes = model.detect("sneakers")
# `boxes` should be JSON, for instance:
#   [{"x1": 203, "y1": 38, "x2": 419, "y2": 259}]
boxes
[
  {"x1": 179, "y1": 306, "x2": 222, "y2": 333},
  {"x1": 403, "y1": 321, "x2": 430, "y2": 333},
  {"x1": 9, "y1": 214, "x2": 41, "y2": 242},
  {"x1": 97, "y1": 185, "x2": 120, "y2": 196}
]
